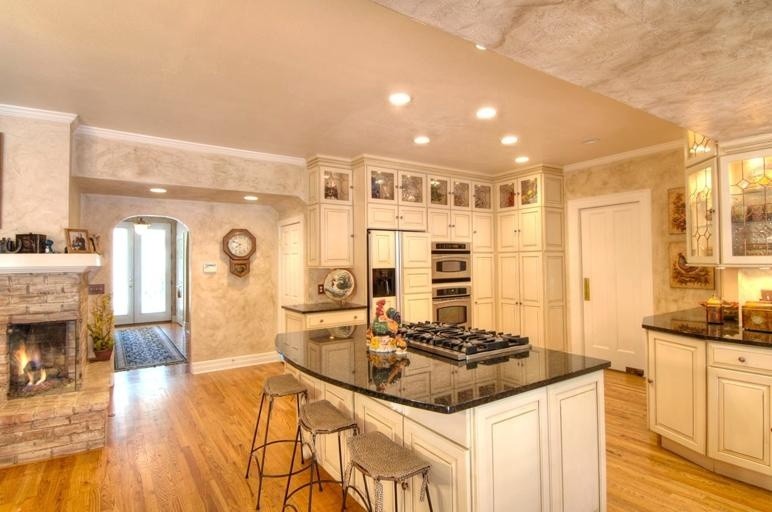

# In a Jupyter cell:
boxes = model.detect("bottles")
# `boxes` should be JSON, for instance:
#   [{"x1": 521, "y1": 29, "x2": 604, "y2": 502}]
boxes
[
  {"x1": 325, "y1": 172, "x2": 337, "y2": 200},
  {"x1": 508, "y1": 192, "x2": 514, "y2": 207},
  {"x1": 372, "y1": 176, "x2": 380, "y2": 198},
  {"x1": 705, "y1": 293, "x2": 724, "y2": 325}
]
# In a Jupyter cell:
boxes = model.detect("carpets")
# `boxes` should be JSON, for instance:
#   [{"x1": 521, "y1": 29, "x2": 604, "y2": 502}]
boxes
[{"x1": 113, "y1": 325, "x2": 187, "y2": 373}]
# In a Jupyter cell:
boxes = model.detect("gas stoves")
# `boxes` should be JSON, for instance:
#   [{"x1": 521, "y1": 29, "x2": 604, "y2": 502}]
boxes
[{"x1": 401, "y1": 321, "x2": 530, "y2": 362}]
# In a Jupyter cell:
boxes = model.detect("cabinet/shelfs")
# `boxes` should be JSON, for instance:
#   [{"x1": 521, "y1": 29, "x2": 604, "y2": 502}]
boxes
[
  {"x1": 308, "y1": 202, "x2": 355, "y2": 271},
  {"x1": 471, "y1": 253, "x2": 497, "y2": 332},
  {"x1": 497, "y1": 253, "x2": 569, "y2": 354},
  {"x1": 284, "y1": 308, "x2": 366, "y2": 375},
  {"x1": 428, "y1": 167, "x2": 471, "y2": 243},
  {"x1": 353, "y1": 391, "x2": 473, "y2": 512},
  {"x1": 471, "y1": 173, "x2": 495, "y2": 253},
  {"x1": 353, "y1": 156, "x2": 428, "y2": 232},
  {"x1": 681, "y1": 129, "x2": 772, "y2": 270},
  {"x1": 706, "y1": 341, "x2": 772, "y2": 492},
  {"x1": 644, "y1": 324, "x2": 707, "y2": 472},
  {"x1": 286, "y1": 329, "x2": 571, "y2": 404},
  {"x1": 496, "y1": 165, "x2": 565, "y2": 253},
  {"x1": 297, "y1": 370, "x2": 354, "y2": 502},
  {"x1": 305, "y1": 155, "x2": 354, "y2": 206}
]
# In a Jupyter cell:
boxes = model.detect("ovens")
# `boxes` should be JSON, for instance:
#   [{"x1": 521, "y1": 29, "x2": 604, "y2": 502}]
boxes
[{"x1": 430, "y1": 254, "x2": 475, "y2": 327}]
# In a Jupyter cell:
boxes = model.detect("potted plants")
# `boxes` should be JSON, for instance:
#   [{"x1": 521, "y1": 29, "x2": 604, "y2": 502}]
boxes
[{"x1": 84, "y1": 291, "x2": 115, "y2": 361}]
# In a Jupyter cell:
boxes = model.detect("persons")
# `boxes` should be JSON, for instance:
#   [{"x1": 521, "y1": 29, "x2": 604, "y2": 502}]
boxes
[{"x1": 73, "y1": 233, "x2": 85, "y2": 250}]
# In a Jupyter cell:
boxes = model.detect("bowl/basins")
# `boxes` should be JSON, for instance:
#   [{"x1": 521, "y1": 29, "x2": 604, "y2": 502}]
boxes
[{"x1": 700, "y1": 302, "x2": 738, "y2": 318}]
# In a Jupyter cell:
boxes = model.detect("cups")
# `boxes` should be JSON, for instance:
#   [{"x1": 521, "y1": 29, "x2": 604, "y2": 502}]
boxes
[{"x1": 732, "y1": 203, "x2": 771, "y2": 221}]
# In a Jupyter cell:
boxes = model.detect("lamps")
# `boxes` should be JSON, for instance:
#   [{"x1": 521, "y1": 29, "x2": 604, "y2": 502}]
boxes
[{"x1": 133, "y1": 217, "x2": 151, "y2": 236}]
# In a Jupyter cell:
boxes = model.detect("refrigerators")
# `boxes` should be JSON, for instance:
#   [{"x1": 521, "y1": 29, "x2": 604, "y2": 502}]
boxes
[{"x1": 367, "y1": 231, "x2": 432, "y2": 324}]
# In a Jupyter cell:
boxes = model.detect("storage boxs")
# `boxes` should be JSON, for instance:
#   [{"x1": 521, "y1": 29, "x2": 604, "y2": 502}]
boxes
[
  {"x1": 15, "y1": 233, "x2": 47, "y2": 253},
  {"x1": 740, "y1": 300, "x2": 771, "y2": 334}
]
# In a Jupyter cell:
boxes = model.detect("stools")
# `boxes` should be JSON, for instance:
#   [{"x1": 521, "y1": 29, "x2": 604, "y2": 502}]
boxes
[
  {"x1": 283, "y1": 399, "x2": 373, "y2": 512},
  {"x1": 340, "y1": 432, "x2": 434, "y2": 512},
  {"x1": 245, "y1": 373, "x2": 323, "y2": 512}
]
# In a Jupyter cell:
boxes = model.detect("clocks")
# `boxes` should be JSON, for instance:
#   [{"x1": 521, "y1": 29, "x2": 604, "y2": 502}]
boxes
[{"x1": 222, "y1": 228, "x2": 256, "y2": 278}]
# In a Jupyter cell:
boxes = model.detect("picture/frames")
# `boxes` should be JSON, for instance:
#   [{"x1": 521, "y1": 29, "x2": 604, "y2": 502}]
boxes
[{"x1": 63, "y1": 227, "x2": 92, "y2": 254}]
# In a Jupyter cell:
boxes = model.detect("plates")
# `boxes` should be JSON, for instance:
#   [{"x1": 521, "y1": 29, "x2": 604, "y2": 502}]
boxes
[{"x1": 324, "y1": 269, "x2": 356, "y2": 300}]
